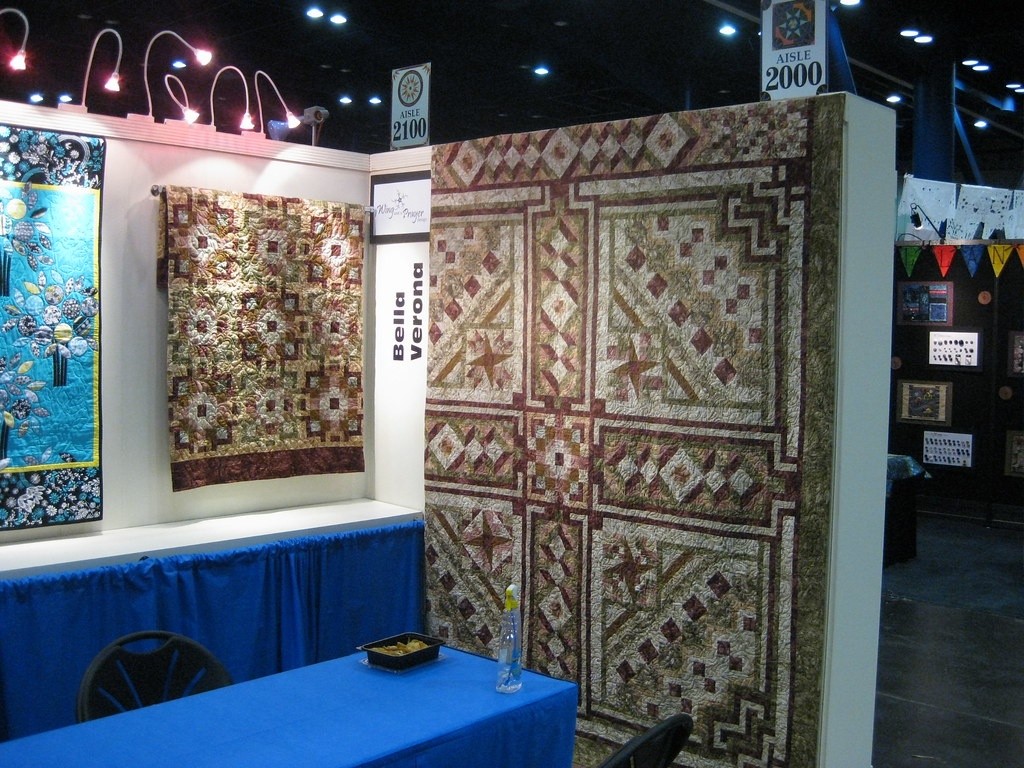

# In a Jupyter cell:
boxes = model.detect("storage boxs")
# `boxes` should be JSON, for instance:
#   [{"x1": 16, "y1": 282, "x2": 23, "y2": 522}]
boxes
[{"x1": 354, "y1": 632, "x2": 449, "y2": 674}]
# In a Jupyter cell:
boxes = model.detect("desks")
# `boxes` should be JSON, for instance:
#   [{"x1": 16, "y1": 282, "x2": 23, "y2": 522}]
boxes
[{"x1": 0, "y1": 646, "x2": 576, "y2": 768}]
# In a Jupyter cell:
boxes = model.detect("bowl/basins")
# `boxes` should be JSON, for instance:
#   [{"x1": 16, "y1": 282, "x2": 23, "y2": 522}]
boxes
[{"x1": 360, "y1": 631, "x2": 446, "y2": 670}]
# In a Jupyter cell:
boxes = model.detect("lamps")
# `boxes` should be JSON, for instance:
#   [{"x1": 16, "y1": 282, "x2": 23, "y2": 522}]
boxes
[
  {"x1": 210, "y1": 66, "x2": 259, "y2": 132},
  {"x1": 82, "y1": 28, "x2": 122, "y2": 106},
  {"x1": 909, "y1": 202, "x2": 947, "y2": 245},
  {"x1": 0, "y1": 9, "x2": 28, "y2": 70},
  {"x1": 144, "y1": 31, "x2": 212, "y2": 115},
  {"x1": 242, "y1": 71, "x2": 302, "y2": 139},
  {"x1": 165, "y1": 74, "x2": 200, "y2": 124}
]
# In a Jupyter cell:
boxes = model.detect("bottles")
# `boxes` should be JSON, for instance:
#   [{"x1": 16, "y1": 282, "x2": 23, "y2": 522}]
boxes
[{"x1": 497, "y1": 584, "x2": 523, "y2": 694}]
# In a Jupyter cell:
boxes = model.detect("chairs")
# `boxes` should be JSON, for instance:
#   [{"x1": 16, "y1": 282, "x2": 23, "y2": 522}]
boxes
[
  {"x1": 73, "y1": 630, "x2": 234, "y2": 724},
  {"x1": 599, "y1": 711, "x2": 695, "y2": 768}
]
